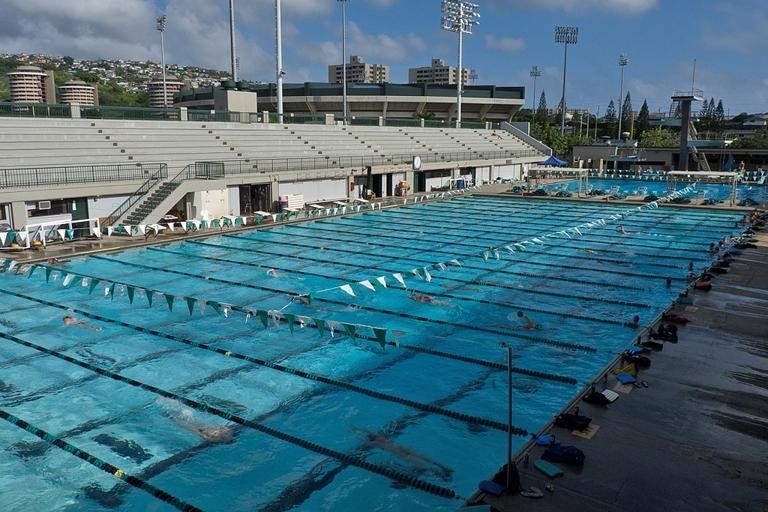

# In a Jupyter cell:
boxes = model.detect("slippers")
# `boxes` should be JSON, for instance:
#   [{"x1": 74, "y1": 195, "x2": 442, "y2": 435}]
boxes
[
  {"x1": 633, "y1": 380, "x2": 649, "y2": 388},
  {"x1": 520, "y1": 482, "x2": 555, "y2": 498}
]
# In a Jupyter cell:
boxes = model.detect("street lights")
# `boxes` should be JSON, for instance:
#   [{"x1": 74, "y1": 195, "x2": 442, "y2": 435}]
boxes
[
  {"x1": 617, "y1": 53, "x2": 629, "y2": 142},
  {"x1": 553, "y1": 23, "x2": 578, "y2": 139},
  {"x1": 691, "y1": 57, "x2": 696, "y2": 95},
  {"x1": 529, "y1": 64, "x2": 540, "y2": 128},
  {"x1": 467, "y1": 68, "x2": 478, "y2": 83},
  {"x1": 497, "y1": 341, "x2": 513, "y2": 488},
  {"x1": 154, "y1": 12, "x2": 167, "y2": 108},
  {"x1": 439, "y1": 0, "x2": 481, "y2": 130}
]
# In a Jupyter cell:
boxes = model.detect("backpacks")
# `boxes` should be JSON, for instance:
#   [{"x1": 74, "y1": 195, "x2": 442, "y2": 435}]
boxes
[{"x1": 559, "y1": 445, "x2": 586, "y2": 467}]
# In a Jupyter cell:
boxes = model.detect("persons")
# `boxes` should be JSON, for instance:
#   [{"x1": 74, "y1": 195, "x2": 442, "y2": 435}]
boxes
[
  {"x1": 437, "y1": 283, "x2": 481, "y2": 293},
  {"x1": 159, "y1": 408, "x2": 240, "y2": 446},
  {"x1": 486, "y1": 311, "x2": 542, "y2": 331},
  {"x1": 627, "y1": 313, "x2": 641, "y2": 328},
  {"x1": 615, "y1": 225, "x2": 627, "y2": 234},
  {"x1": 266, "y1": 269, "x2": 303, "y2": 281},
  {"x1": 712, "y1": 246, "x2": 720, "y2": 254},
  {"x1": 410, "y1": 292, "x2": 454, "y2": 307},
  {"x1": 354, "y1": 421, "x2": 456, "y2": 485},
  {"x1": 726, "y1": 207, "x2": 765, "y2": 246},
  {"x1": 687, "y1": 260, "x2": 693, "y2": 271},
  {"x1": 709, "y1": 242, "x2": 716, "y2": 250},
  {"x1": 581, "y1": 248, "x2": 598, "y2": 254},
  {"x1": 719, "y1": 241, "x2": 727, "y2": 250},
  {"x1": 289, "y1": 296, "x2": 327, "y2": 313},
  {"x1": 665, "y1": 277, "x2": 673, "y2": 287},
  {"x1": 61, "y1": 314, "x2": 105, "y2": 336}
]
